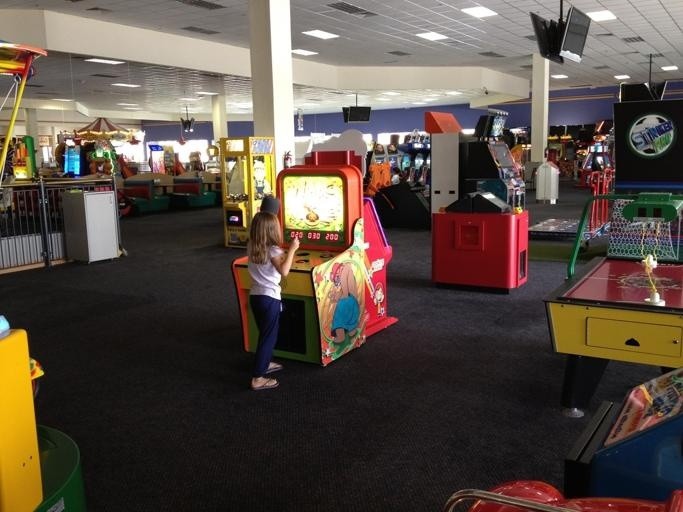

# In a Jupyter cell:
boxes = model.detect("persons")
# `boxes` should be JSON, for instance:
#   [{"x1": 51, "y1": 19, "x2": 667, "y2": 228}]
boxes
[
  {"x1": 259, "y1": 195, "x2": 280, "y2": 214},
  {"x1": 247, "y1": 210, "x2": 301, "y2": 392}
]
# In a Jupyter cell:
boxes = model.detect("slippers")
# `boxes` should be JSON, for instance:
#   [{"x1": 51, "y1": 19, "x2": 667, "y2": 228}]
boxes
[
  {"x1": 265, "y1": 363, "x2": 283, "y2": 374},
  {"x1": 252, "y1": 377, "x2": 279, "y2": 391}
]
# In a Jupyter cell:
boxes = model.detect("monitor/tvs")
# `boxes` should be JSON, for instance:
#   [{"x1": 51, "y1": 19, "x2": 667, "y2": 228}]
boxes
[
  {"x1": 374, "y1": 152, "x2": 431, "y2": 174},
  {"x1": 529, "y1": 6, "x2": 591, "y2": 66},
  {"x1": 621, "y1": 80, "x2": 669, "y2": 101},
  {"x1": 342, "y1": 106, "x2": 371, "y2": 123},
  {"x1": 493, "y1": 145, "x2": 514, "y2": 164},
  {"x1": 282, "y1": 175, "x2": 344, "y2": 232},
  {"x1": 181, "y1": 117, "x2": 195, "y2": 132}
]
